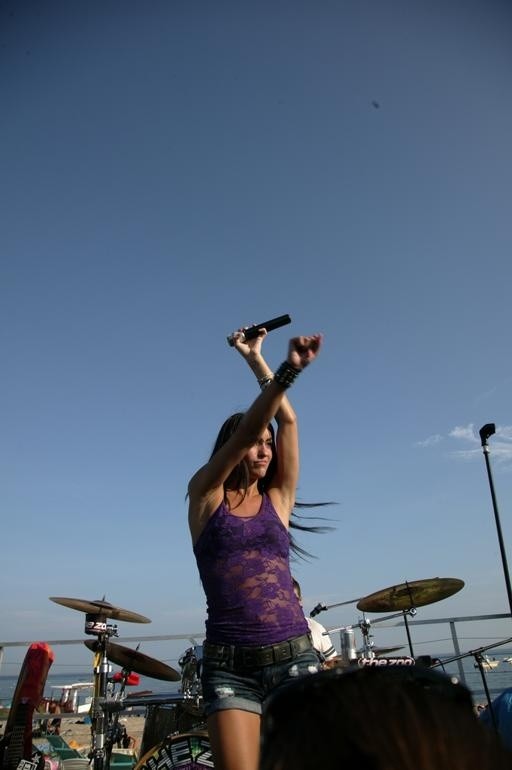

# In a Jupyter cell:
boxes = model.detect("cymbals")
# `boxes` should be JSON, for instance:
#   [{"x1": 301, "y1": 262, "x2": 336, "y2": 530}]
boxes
[
  {"x1": 356, "y1": 578, "x2": 464, "y2": 612},
  {"x1": 50, "y1": 598, "x2": 150, "y2": 624},
  {"x1": 355, "y1": 645, "x2": 401, "y2": 653},
  {"x1": 85, "y1": 639, "x2": 180, "y2": 682}
]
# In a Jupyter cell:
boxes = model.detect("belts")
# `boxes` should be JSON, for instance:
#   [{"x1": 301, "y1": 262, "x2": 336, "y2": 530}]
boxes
[{"x1": 202, "y1": 632, "x2": 314, "y2": 669}]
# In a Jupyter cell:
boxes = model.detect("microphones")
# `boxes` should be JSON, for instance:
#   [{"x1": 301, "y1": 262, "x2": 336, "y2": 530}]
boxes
[
  {"x1": 309, "y1": 600, "x2": 326, "y2": 618},
  {"x1": 226, "y1": 314, "x2": 292, "y2": 348}
]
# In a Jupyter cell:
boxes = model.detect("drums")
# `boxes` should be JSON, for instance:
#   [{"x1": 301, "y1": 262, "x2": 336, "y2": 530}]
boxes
[{"x1": 140, "y1": 645, "x2": 207, "y2": 761}]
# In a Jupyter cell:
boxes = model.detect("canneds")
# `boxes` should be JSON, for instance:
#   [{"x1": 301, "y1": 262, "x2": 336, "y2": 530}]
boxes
[{"x1": 340, "y1": 628, "x2": 357, "y2": 661}]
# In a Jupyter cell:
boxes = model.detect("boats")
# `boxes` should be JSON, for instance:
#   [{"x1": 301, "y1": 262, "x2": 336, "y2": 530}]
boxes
[{"x1": 473, "y1": 655, "x2": 512, "y2": 669}]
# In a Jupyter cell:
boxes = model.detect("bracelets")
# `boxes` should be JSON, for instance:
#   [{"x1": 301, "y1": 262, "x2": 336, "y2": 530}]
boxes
[
  {"x1": 273, "y1": 361, "x2": 304, "y2": 388},
  {"x1": 258, "y1": 372, "x2": 272, "y2": 391}
]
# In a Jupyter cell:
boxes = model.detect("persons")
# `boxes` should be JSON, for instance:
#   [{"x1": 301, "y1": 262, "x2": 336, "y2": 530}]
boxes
[
  {"x1": 51, "y1": 702, "x2": 62, "y2": 733},
  {"x1": 187, "y1": 323, "x2": 329, "y2": 770},
  {"x1": 292, "y1": 578, "x2": 342, "y2": 666}
]
